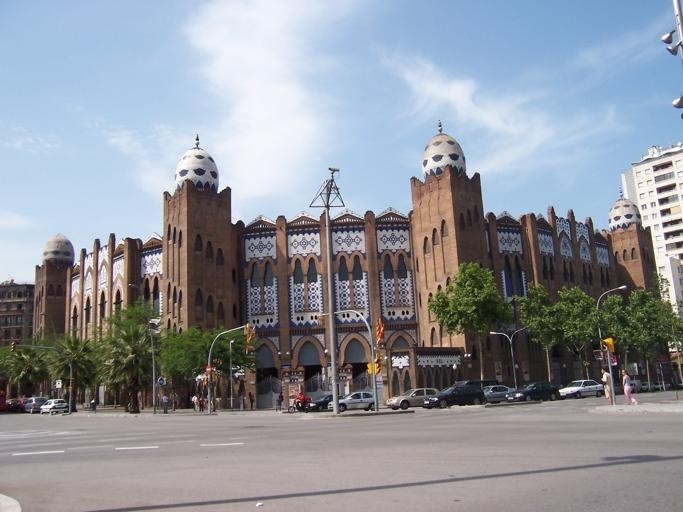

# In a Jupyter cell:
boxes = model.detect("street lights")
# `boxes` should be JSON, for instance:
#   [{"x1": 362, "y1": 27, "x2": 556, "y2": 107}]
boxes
[
  {"x1": 488, "y1": 321, "x2": 539, "y2": 389},
  {"x1": 596, "y1": 285, "x2": 627, "y2": 370},
  {"x1": 660, "y1": 1, "x2": 682, "y2": 119},
  {"x1": 228, "y1": 339, "x2": 235, "y2": 408},
  {"x1": 316, "y1": 309, "x2": 380, "y2": 411}
]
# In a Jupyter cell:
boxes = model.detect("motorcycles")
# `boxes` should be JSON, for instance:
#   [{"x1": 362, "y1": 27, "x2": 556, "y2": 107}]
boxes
[{"x1": 287, "y1": 397, "x2": 314, "y2": 413}]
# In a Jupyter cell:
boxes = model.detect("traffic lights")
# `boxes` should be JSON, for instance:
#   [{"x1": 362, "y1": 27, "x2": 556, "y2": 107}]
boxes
[
  {"x1": 598, "y1": 336, "x2": 614, "y2": 353},
  {"x1": 10, "y1": 341, "x2": 15, "y2": 352},
  {"x1": 374, "y1": 358, "x2": 381, "y2": 375},
  {"x1": 366, "y1": 362, "x2": 372, "y2": 374}
]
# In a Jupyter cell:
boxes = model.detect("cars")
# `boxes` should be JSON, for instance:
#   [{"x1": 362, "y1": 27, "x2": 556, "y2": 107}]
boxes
[
  {"x1": 327, "y1": 391, "x2": 374, "y2": 412},
  {"x1": 481, "y1": 385, "x2": 516, "y2": 404},
  {"x1": 422, "y1": 384, "x2": 484, "y2": 409},
  {"x1": 504, "y1": 380, "x2": 561, "y2": 402},
  {"x1": 5, "y1": 396, "x2": 68, "y2": 415},
  {"x1": 556, "y1": 379, "x2": 604, "y2": 400},
  {"x1": 385, "y1": 387, "x2": 439, "y2": 410},
  {"x1": 613, "y1": 379, "x2": 683, "y2": 395},
  {"x1": 304, "y1": 394, "x2": 345, "y2": 412}
]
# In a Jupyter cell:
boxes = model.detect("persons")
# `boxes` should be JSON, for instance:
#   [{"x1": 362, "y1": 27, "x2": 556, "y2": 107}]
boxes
[
  {"x1": 296, "y1": 393, "x2": 305, "y2": 408},
  {"x1": 600, "y1": 367, "x2": 612, "y2": 405},
  {"x1": 239, "y1": 393, "x2": 244, "y2": 410},
  {"x1": 162, "y1": 392, "x2": 205, "y2": 413},
  {"x1": 279, "y1": 392, "x2": 284, "y2": 408},
  {"x1": 622, "y1": 369, "x2": 639, "y2": 405},
  {"x1": 249, "y1": 392, "x2": 255, "y2": 410}
]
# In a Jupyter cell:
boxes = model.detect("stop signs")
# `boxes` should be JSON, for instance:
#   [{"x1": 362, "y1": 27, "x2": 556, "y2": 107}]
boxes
[{"x1": 205, "y1": 367, "x2": 211, "y2": 373}]
